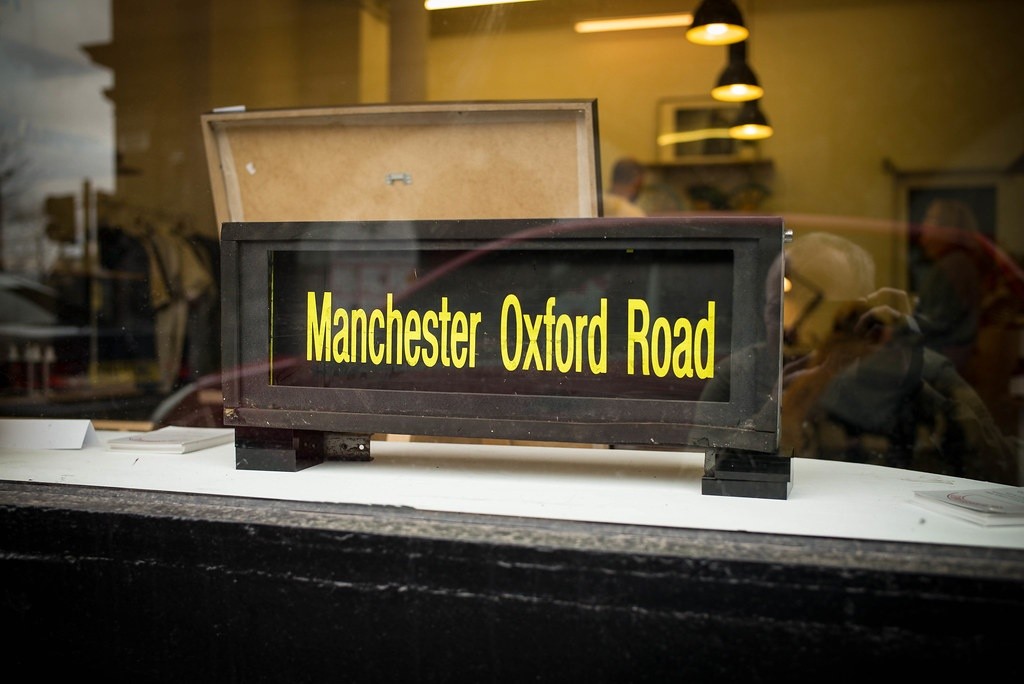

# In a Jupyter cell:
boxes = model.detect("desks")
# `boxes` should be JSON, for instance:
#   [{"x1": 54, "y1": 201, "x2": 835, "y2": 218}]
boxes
[{"x1": 1, "y1": 424, "x2": 1024, "y2": 684}]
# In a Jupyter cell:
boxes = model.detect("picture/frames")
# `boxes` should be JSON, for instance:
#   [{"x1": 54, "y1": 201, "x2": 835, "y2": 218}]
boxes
[
  {"x1": 657, "y1": 97, "x2": 759, "y2": 162},
  {"x1": 888, "y1": 168, "x2": 1004, "y2": 304}
]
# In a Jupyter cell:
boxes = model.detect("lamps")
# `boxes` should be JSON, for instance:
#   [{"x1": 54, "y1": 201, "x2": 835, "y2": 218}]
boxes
[
  {"x1": 685, "y1": 0, "x2": 751, "y2": 48},
  {"x1": 709, "y1": 40, "x2": 764, "y2": 102},
  {"x1": 730, "y1": 100, "x2": 772, "y2": 141}
]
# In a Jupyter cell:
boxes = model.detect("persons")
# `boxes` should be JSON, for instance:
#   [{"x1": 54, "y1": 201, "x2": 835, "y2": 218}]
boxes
[
  {"x1": 684, "y1": 233, "x2": 1004, "y2": 482},
  {"x1": 601, "y1": 160, "x2": 647, "y2": 217},
  {"x1": 920, "y1": 199, "x2": 1024, "y2": 438}
]
